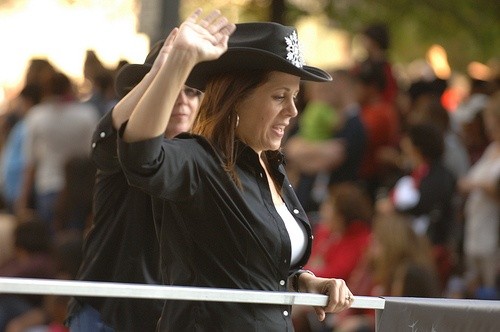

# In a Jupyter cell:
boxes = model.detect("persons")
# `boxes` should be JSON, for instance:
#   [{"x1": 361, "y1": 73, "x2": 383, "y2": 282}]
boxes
[
  {"x1": 0, "y1": 47, "x2": 128, "y2": 332},
  {"x1": 280, "y1": 19, "x2": 498, "y2": 331},
  {"x1": 63, "y1": 28, "x2": 210, "y2": 332},
  {"x1": 116, "y1": 6, "x2": 352, "y2": 332}
]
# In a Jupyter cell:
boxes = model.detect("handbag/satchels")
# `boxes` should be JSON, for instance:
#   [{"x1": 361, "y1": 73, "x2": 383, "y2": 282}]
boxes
[{"x1": 17, "y1": 209, "x2": 55, "y2": 278}]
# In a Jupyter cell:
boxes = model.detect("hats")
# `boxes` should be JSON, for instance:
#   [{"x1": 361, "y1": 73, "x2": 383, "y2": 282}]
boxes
[
  {"x1": 84, "y1": 51, "x2": 128, "y2": 85},
  {"x1": 114, "y1": 40, "x2": 164, "y2": 96},
  {"x1": 21, "y1": 59, "x2": 70, "y2": 95},
  {"x1": 184, "y1": 21, "x2": 334, "y2": 82}
]
